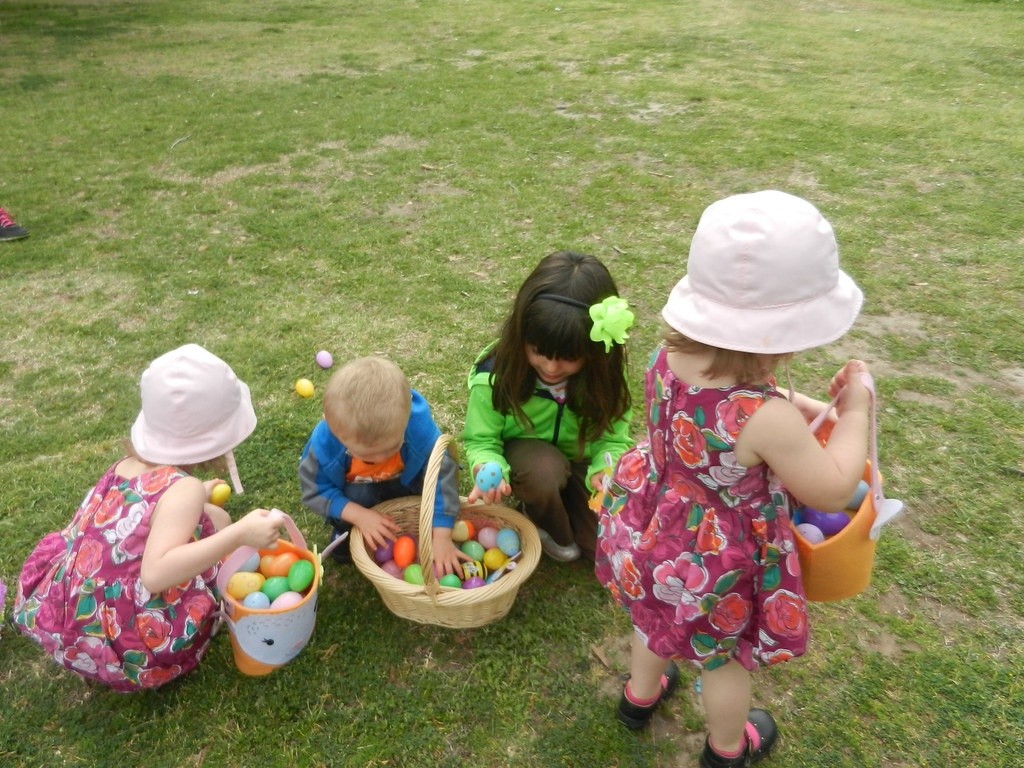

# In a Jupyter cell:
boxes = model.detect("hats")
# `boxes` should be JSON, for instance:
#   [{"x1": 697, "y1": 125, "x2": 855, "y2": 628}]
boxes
[
  {"x1": 661, "y1": 189, "x2": 866, "y2": 354},
  {"x1": 130, "y1": 343, "x2": 257, "y2": 465}
]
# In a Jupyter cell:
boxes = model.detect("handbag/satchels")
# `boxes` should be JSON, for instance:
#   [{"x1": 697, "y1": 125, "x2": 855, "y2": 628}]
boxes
[
  {"x1": 788, "y1": 373, "x2": 903, "y2": 601},
  {"x1": 215, "y1": 510, "x2": 323, "y2": 676}
]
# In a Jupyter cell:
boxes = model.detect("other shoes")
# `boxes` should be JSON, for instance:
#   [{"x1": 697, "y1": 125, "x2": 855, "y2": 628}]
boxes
[
  {"x1": 0, "y1": 206, "x2": 31, "y2": 240},
  {"x1": 330, "y1": 524, "x2": 354, "y2": 563},
  {"x1": 525, "y1": 515, "x2": 580, "y2": 561},
  {"x1": 700, "y1": 708, "x2": 778, "y2": 768},
  {"x1": 616, "y1": 658, "x2": 678, "y2": 727}
]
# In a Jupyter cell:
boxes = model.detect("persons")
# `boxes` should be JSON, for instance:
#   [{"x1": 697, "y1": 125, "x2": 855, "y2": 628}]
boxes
[
  {"x1": 16, "y1": 343, "x2": 282, "y2": 695},
  {"x1": 597, "y1": 187, "x2": 872, "y2": 768},
  {"x1": 461, "y1": 251, "x2": 635, "y2": 564},
  {"x1": 298, "y1": 357, "x2": 473, "y2": 581}
]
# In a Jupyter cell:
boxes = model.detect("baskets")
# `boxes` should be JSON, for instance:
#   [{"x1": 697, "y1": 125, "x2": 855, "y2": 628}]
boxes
[{"x1": 350, "y1": 432, "x2": 541, "y2": 628}]
